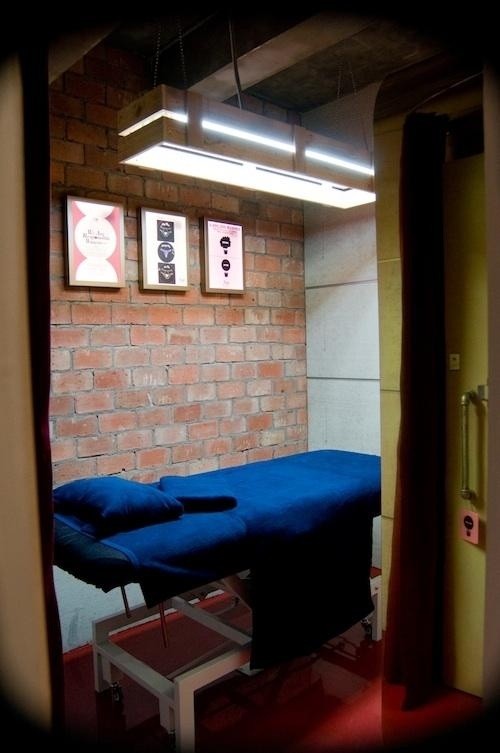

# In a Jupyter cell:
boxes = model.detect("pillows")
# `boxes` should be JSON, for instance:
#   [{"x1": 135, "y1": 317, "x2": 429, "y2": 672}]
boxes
[{"x1": 52, "y1": 472, "x2": 185, "y2": 533}]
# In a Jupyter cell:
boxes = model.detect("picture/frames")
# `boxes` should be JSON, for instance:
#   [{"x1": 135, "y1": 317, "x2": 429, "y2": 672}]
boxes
[
  {"x1": 137, "y1": 207, "x2": 190, "y2": 292},
  {"x1": 64, "y1": 194, "x2": 127, "y2": 289},
  {"x1": 199, "y1": 214, "x2": 247, "y2": 296}
]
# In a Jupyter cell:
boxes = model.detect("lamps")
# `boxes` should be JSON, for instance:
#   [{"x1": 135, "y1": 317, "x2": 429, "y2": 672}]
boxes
[{"x1": 116, "y1": 3, "x2": 378, "y2": 211}]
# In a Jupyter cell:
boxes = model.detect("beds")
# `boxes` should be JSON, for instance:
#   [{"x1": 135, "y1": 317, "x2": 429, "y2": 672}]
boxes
[{"x1": 51, "y1": 450, "x2": 384, "y2": 751}]
